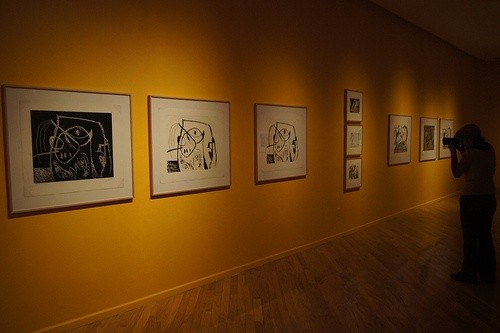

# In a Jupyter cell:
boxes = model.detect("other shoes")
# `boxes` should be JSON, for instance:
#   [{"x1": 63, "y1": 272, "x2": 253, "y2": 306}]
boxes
[
  {"x1": 480, "y1": 275, "x2": 496, "y2": 284},
  {"x1": 450, "y1": 273, "x2": 471, "y2": 281}
]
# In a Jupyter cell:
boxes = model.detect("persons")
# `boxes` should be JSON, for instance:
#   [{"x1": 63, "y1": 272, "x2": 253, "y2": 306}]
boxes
[{"x1": 450, "y1": 125, "x2": 496, "y2": 282}]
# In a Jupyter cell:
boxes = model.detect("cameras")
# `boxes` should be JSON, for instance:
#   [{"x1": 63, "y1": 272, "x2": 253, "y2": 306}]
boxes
[{"x1": 443, "y1": 132, "x2": 463, "y2": 150}]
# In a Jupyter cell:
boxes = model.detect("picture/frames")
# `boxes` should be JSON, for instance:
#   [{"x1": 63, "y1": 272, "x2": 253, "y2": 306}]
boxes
[
  {"x1": 344, "y1": 88, "x2": 363, "y2": 123},
  {"x1": 147, "y1": 95, "x2": 231, "y2": 200},
  {"x1": 438, "y1": 118, "x2": 454, "y2": 160},
  {"x1": 344, "y1": 123, "x2": 362, "y2": 157},
  {"x1": 343, "y1": 157, "x2": 362, "y2": 193},
  {"x1": 419, "y1": 117, "x2": 438, "y2": 163},
  {"x1": 387, "y1": 114, "x2": 412, "y2": 166},
  {"x1": 254, "y1": 103, "x2": 307, "y2": 185},
  {"x1": 0, "y1": 84, "x2": 134, "y2": 219}
]
